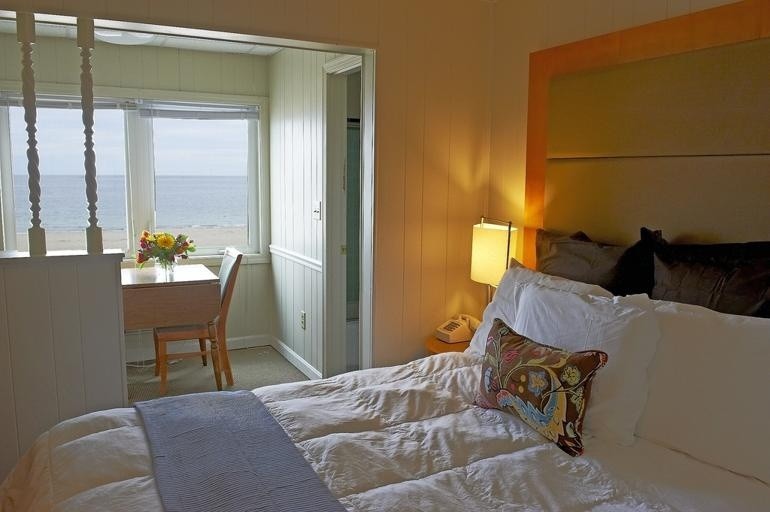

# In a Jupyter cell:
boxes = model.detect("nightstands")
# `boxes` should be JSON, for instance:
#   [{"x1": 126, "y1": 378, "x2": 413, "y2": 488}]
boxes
[{"x1": 422, "y1": 332, "x2": 470, "y2": 356}]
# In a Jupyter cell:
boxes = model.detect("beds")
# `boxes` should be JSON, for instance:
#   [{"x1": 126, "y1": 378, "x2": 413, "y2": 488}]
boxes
[{"x1": 0, "y1": 351, "x2": 770, "y2": 512}]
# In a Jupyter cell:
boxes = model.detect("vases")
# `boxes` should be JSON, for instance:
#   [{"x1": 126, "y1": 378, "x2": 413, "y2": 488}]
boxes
[{"x1": 155, "y1": 256, "x2": 177, "y2": 273}]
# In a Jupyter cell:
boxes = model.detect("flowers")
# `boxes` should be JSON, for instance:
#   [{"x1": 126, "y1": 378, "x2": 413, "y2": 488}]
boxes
[{"x1": 130, "y1": 229, "x2": 197, "y2": 272}]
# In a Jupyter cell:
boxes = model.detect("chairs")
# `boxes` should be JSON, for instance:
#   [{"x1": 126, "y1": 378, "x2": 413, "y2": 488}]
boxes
[{"x1": 153, "y1": 246, "x2": 243, "y2": 397}]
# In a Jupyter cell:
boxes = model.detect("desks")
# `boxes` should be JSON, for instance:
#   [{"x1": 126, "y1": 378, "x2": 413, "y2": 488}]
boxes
[{"x1": 121, "y1": 263, "x2": 223, "y2": 392}]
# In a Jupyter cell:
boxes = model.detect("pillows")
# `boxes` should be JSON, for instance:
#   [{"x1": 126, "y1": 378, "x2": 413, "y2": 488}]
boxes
[
  {"x1": 471, "y1": 318, "x2": 609, "y2": 457},
  {"x1": 633, "y1": 298, "x2": 769, "y2": 484},
  {"x1": 467, "y1": 258, "x2": 612, "y2": 356},
  {"x1": 510, "y1": 279, "x2": 678, "y2": 445}
]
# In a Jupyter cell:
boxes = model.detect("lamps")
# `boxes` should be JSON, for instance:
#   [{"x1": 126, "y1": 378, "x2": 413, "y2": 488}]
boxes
[{"x1": 470, "y1": 215, "x2": 519, "y2": 305}]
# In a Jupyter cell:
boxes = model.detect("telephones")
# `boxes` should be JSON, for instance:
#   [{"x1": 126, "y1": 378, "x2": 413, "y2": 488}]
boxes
[{"x1": 433, "y1": 313, "x2": 481, "y2": 343}]
